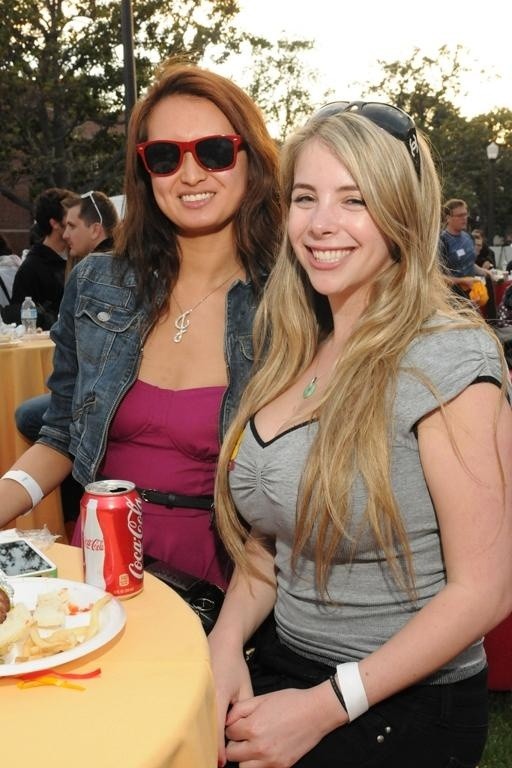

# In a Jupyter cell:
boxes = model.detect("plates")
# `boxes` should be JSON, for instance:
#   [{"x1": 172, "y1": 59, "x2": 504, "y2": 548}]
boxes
[
  {"x1": 0, "y1": 575, "x2": 128, "y2": 679},
  {"x1": 0, "y1": 338, "x2": 20, "y2": 350}
]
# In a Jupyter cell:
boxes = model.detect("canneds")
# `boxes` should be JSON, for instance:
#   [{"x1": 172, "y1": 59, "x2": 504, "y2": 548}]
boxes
[{"x1": 78, "y1": 479, "x2": 145, "y2": 602}]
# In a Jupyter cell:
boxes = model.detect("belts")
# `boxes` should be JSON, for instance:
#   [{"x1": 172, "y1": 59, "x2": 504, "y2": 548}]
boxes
[{"x1": 135, "y1": 487, "x2": 214, "y2": 509}]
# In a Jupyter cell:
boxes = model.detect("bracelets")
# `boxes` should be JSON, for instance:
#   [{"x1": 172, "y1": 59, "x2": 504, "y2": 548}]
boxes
[
  {"x1": 329, "y1": 676, "x2": 348, "y2": 713},
  {"x1": 1, "y1": 470, "x2": 44, "y2": 515},
  {"x1": 336, "y1": 662, "x2": 369, "y2": 723}
]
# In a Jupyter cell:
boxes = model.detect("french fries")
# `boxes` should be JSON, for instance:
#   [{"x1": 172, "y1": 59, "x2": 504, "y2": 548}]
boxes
[{"x1": 14, "y1": 594, "x2": 113, "y2": 661}]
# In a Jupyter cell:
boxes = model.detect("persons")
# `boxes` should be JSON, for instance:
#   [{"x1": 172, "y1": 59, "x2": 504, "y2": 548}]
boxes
[
  {"x1": 208, "y1": 100, "x2": 512, "y2": 768},
  {"x1": 0, "y1": 55, "x2": 282, "y2": 637},
  {"x1": 1, "y1": 188, "x2": 121, "y2": 443},
  {"x1": 437, "y1": 199, "x2": 512, "y2": 382}
]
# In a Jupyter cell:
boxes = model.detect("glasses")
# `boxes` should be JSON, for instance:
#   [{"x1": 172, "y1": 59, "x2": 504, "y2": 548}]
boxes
[
  {"x1": 136, "y1": 135, "x2": 243, "y2": 177},
  {"x1": 305, "y1": 101, "x2": 420, "y2": 180},
  {"x1": 81, "y1": 191, "x2": 103, "y2": 225}
]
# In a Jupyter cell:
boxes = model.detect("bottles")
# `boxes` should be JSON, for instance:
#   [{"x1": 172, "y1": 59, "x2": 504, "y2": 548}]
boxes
[{"x1": 20, "y1": 294, "x2": 38, "y2": 336}]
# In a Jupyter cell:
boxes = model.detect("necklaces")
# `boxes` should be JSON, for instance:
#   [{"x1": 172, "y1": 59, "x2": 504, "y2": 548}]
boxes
[
  {"x1": 173, "y1": 267, "x2": 241, "y2": 342},
  {"x1": 303, "y1": 354, "x2": 337, "y2": 399}
]
